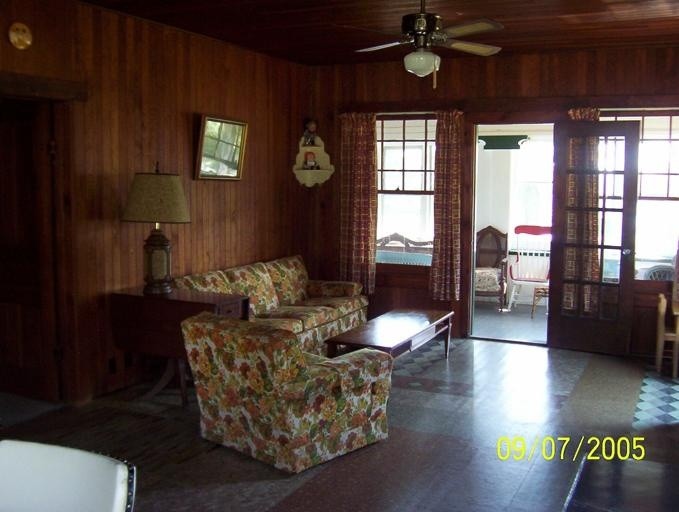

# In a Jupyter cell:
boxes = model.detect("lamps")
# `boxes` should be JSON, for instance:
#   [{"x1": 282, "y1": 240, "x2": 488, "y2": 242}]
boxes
[
  {"x1": 120, "y1": 171, "x2": 191, "y2": 296},
  {"x1": 403, "y1": 51, "x2": 442, "y2": 76}
]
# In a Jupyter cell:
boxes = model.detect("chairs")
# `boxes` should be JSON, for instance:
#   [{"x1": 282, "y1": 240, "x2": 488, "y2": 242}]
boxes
[
  {"x1": 179, "y1": 310, "x2": 394, "y2": 475},
  {"x1": 475, "y1": 226, "x2": 508, "y2": 312},
  {"x1": 654, "y1": 292, "x2": 674, "y2": 372},
  {"x1": 508, "y1": 225, "x2": 552, "y2": 312},
  {"x1": 643, "y1": 265, "x2": 675, "y2": 281},
  {"x1": 0, "y1": 440, "x2": 136, "y2": 512}
]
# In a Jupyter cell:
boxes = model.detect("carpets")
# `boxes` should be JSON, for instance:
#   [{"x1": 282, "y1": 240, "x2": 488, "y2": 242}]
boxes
[{"x1": 630, "y1": 361, "x2": 679, "y2": 433}]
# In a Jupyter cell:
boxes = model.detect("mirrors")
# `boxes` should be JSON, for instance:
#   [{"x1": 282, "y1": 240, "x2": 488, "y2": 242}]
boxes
[{"x1": 194, "y1": 113, "x2": 248, "y2": 181}]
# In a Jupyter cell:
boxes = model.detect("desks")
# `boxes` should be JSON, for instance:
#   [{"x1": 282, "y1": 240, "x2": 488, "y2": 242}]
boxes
[
  {"x1": 531, "y1": 287, "x2": 572, "y2": 321},
  {"x1": 669, "y1": 298, "x2": 679, "y2": 378},
  {"x1": 561, "y1": 451, "x2": 678, "y2": 512}
]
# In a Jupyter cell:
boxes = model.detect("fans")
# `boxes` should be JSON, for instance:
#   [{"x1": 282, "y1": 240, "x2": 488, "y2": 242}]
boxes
[{"x1": 354, "y1": 0, "x2": 503, "y2": 58}]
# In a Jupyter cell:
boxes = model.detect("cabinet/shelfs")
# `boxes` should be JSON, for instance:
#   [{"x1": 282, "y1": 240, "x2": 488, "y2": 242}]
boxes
[{"x1": 108, "y1": 287, "x2": 249, "y2": 405}]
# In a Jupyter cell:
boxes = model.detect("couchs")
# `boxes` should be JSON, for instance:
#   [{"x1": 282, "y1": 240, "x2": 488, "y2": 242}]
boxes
[
  {"x1": 375, "y1": 233, "x2": 433, "y2": 266},
  {"x1": 172, "y1": 254, "x2": 370, "y2": 358}
]
